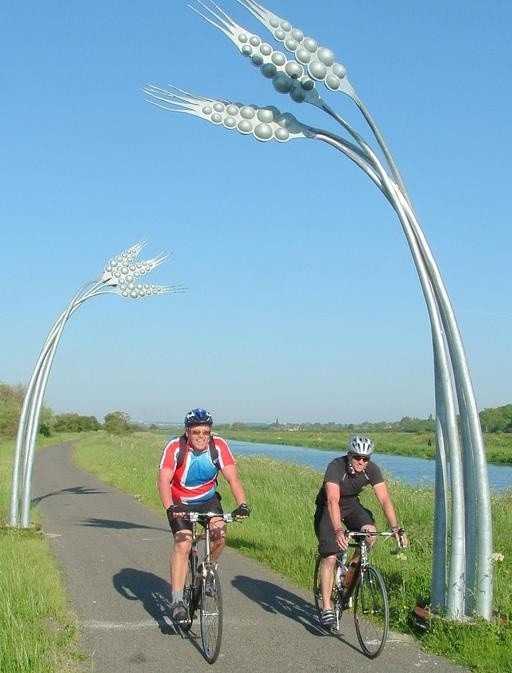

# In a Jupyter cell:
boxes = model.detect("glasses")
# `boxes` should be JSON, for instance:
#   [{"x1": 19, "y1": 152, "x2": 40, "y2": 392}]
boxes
[
  {"x1": 191, "y1": 430, "x2": 210, "y2": 436},
  {"x1": 350, "y1": 453, "x2": 369, "y2": 462}
]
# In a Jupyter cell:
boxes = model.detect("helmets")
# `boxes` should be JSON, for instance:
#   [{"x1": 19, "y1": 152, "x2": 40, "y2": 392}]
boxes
[
  {"x1": 349, "y1": 436, "x2": 375, "y2": 457},
  {"x1": 184, "y1": 408, "x2": 213, "y2": 427}
]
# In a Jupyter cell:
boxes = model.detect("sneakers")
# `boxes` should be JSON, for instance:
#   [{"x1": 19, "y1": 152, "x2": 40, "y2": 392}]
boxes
[
  {"x1": 172, "y1": 601, "x2": 192, "y2": 626},
  {"x1": 204, "y1": 572, "x2": 217, "y2": 598},
  {"x1": 319, "y1": 609, "x2": 337, "y2": 630}
]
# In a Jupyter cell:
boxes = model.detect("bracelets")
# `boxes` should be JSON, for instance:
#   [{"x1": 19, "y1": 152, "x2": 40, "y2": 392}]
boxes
[{"x1": 334, "y1": 526, "x2": 345, "y2": 534}]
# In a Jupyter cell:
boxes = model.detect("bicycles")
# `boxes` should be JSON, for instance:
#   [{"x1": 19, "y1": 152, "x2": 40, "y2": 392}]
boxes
[
  {"x1": 172, "y1": 507, "x2": 248, "y2": 666},
  {"x1": 311, "y1": 525, "x2": 408, "y2": 660}
]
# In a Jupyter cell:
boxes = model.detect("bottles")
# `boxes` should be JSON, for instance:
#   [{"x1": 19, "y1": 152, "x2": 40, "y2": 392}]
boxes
[
  {"x1": 343, "y1": 562, "x2": 357, "y2": 587},
  {"x1": 193, "y1": 564, "x2": 202, "y2": 586},
  {"x1": 335, "y1": 564, "x2": 344, "y2": 591}
]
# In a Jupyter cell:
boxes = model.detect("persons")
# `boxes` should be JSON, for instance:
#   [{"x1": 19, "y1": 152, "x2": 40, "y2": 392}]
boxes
[
  {"x1": 156, "y1": 406, "x2": 250, "y2": 627},
  {"x1": 313, "y1": 435, "x2": 409, "y2": 629}
]
